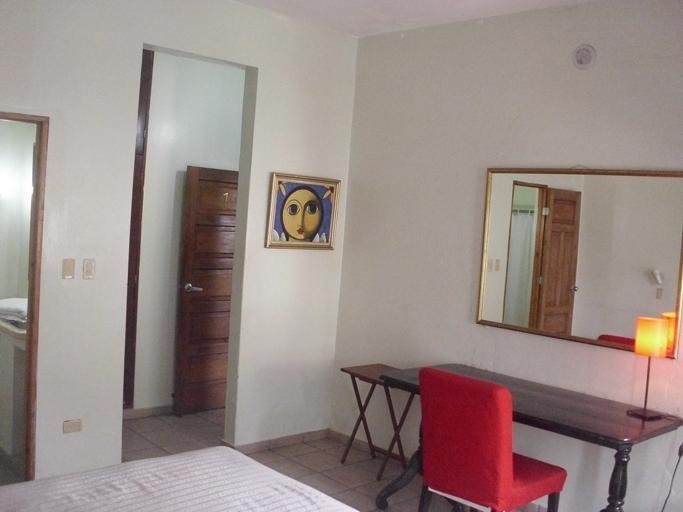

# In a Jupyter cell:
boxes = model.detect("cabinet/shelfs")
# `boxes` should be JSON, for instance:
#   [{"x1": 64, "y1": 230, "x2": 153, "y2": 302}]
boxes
[{"x1": 174, "y1": 166, "x2": 238, "y2": 417}]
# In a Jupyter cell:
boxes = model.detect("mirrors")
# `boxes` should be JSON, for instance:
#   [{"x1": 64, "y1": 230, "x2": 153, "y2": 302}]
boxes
[{"x1": 477, "y1": 168, "x2": 682, "y2": 361}]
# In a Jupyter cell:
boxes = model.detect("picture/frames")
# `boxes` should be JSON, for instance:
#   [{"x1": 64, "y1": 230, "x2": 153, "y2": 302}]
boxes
[{"x1": 265, "y1": 170, "x2": 341, "y2": 250}]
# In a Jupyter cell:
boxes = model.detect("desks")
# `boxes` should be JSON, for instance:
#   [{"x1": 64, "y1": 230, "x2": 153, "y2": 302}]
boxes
[
  {"x1": 340, "y1": 363, "x2": 416, "y2": 483},
  {"x1": 379, "y1": 363, "x2": 682, "y2": 512}
]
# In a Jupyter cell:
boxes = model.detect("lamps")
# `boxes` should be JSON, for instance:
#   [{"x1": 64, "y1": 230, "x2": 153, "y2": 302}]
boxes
[{"x1": 626, "y1": 315, "x2": 669, "y2": 422}]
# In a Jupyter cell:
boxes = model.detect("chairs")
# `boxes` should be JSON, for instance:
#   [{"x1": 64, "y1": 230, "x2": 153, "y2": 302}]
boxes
[{"x1": 417, "y1": 367, "x2": 568, "y2": 512}]
